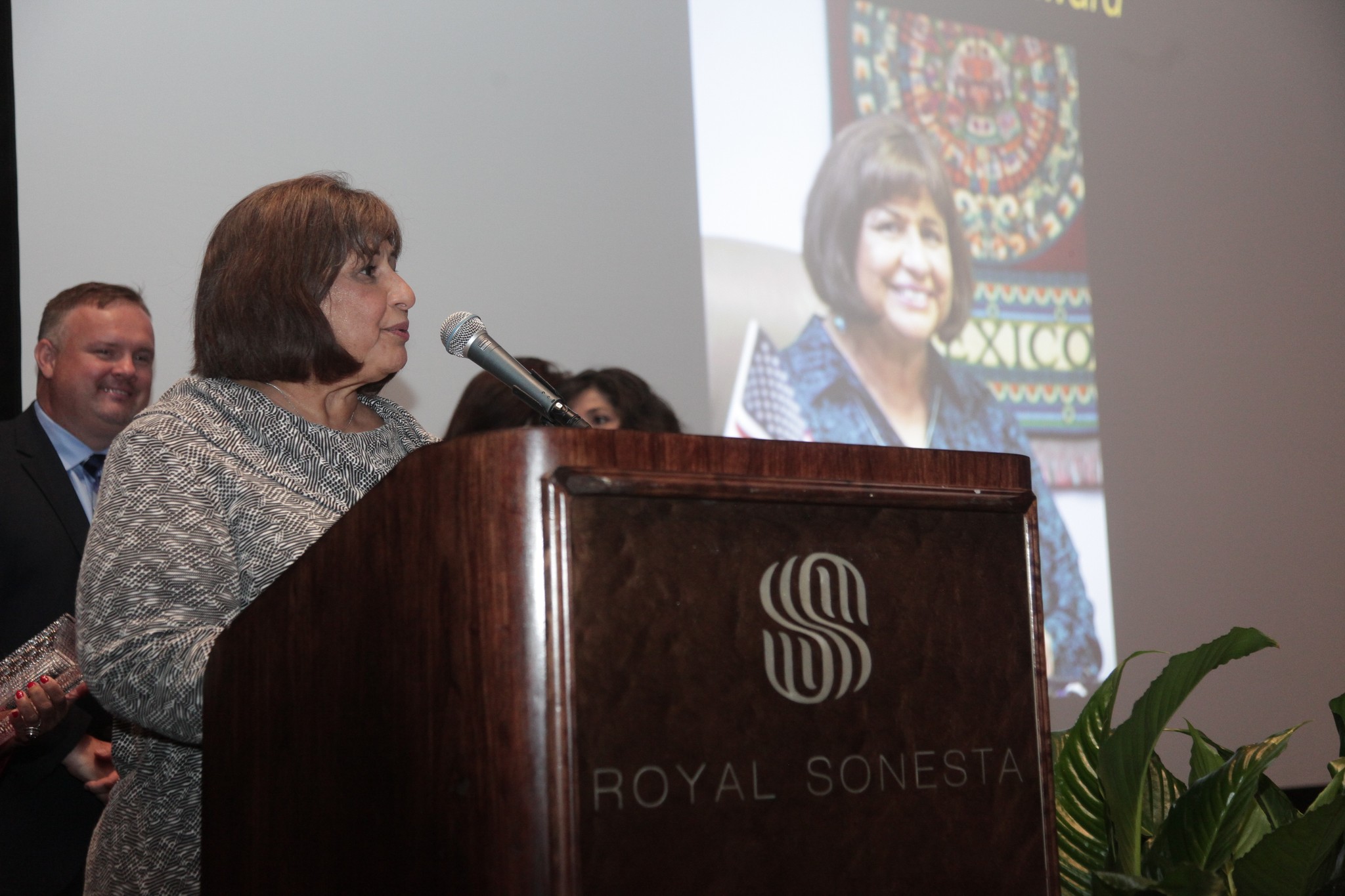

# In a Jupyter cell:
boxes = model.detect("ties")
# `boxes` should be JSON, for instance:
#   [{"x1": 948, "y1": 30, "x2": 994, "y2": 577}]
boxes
[{"x1": 83, "y1": 454, "x2": 104, "y2": 490}]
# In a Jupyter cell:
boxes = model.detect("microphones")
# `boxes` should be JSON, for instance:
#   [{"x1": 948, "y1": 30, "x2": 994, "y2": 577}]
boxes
[{"x1": 441, "y1": 311, "x2": 593, "y2": 428}]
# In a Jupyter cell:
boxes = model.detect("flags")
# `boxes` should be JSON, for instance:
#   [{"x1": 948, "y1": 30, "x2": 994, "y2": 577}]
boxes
[{"x1": 724, "y1": 321, "x2": 817, "y2": 442}]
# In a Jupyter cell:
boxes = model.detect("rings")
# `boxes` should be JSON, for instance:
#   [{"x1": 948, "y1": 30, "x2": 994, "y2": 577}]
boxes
[{"x1": 27, "y1": 718, "x2": 42, "y2": 741}]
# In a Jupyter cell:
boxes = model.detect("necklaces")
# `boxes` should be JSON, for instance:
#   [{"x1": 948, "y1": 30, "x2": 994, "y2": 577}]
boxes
[{"x1": 264, "y1": 382, "x2": 355, "y2": 432}]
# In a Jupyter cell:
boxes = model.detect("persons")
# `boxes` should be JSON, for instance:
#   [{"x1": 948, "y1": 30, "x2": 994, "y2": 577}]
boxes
[
  {"x1": 0, "y1": 279, "x2": 156, "y2": 895},
  {"x1": 76, "y1": 172, "x2": 444, "y2": 894},
  {"x1": 1, "y1": 678, "x2": 87, "y2": 746},
  {"x1": 783, "y1": 117, "x2": 1101, "y2": 695},
  {"x1": 564, "y1": 367, "x2": 682, "y2": 433},
  {"x1": 442, "y1": 356, "x2": 576, "y2": 440}
]
